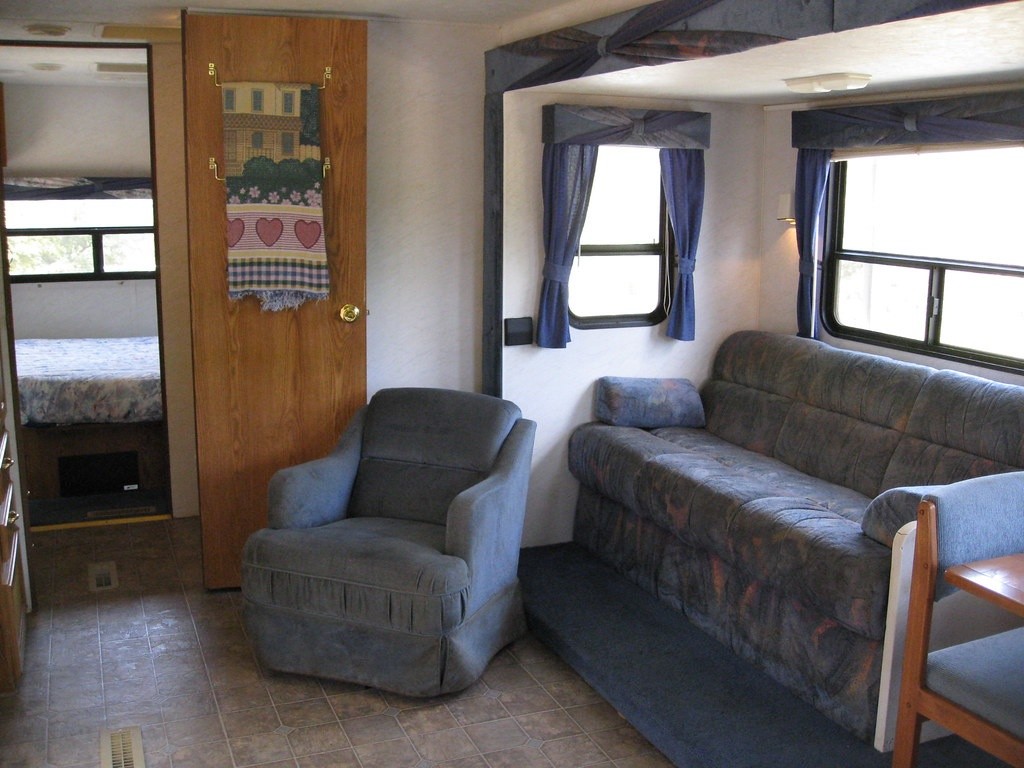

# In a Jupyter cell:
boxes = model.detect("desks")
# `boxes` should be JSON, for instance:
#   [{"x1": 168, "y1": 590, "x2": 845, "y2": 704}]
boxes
[{"x1": 943, "y1": 552, "x2": 1024, "y2": 618}]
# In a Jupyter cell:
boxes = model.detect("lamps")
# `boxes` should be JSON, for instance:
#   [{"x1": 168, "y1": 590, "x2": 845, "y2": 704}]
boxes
[{"x1": 783, "y1": 71, "x2": 873, "y2": 93}]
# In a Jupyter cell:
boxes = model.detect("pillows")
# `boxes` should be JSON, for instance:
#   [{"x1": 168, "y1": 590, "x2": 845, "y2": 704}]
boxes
[
  {"x1": 592, "y1": 373, "x2": 706, "y2": 430},
  {"x1": 857, "y1": 485, "x2": 947, "y2": 544}
]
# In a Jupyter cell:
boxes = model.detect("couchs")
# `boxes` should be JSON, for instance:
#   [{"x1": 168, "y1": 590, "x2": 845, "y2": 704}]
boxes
[
  {"x1": 235, "y1": 387, "x2": 537, "y2": 691},
  {"x1": 563, "y1": 329, "x2": 1024, "y2": 743}
]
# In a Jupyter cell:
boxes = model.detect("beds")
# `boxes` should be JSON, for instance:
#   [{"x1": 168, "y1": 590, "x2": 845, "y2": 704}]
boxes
[{"x1": 17, "y1": 337, "x2": 164, "y2": 496}]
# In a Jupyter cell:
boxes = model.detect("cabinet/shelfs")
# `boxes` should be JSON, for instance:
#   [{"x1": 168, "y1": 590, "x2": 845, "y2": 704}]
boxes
[{"x1": 0, "y1": 389, "x2": 29, "y2": 698}]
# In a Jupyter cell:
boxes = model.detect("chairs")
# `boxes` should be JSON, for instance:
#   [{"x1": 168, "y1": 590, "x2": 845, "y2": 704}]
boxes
[{"x1": 890, "y1": 470, "x2": 1024, "y2": 768}]
